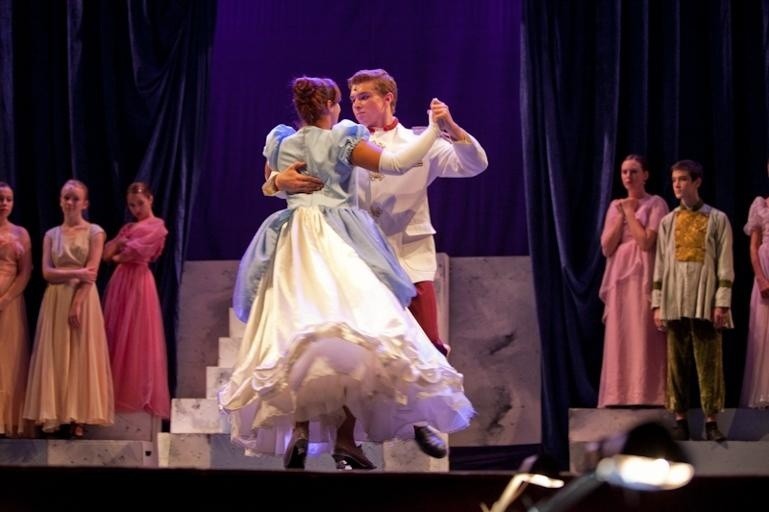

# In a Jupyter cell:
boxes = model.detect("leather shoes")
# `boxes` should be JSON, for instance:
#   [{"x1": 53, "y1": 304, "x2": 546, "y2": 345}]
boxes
[
  {"x1": 705, "y1": 421, "x2": 726, "y2": 443},
  {"x1": 674, "y1": 418, "x2": 690, "y2": 442},
  {"x1": 412, "y1": 424, "x2": 448, "y2": 459}
]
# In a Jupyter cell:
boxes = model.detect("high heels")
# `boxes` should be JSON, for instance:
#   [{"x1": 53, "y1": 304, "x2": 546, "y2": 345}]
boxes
[
  {"x1": 282, "y1": 425, "x2": 309, "y2": 470},
  {"x1": 333, "y1": 442, "x2": 375, "y2": 471}
]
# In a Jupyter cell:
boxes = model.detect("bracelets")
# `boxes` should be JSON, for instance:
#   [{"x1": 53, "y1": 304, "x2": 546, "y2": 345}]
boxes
[{"x1": 270, "y1": 175, "x2": 280, "y2": 193}]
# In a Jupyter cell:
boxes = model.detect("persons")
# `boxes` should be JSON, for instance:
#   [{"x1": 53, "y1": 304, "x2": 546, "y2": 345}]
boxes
[
  {"x1": 737, "y1": 195, "x2": 769, "y2": 412},
  {"x1": 260, "y1": 68, "x2": 491, "y2": 459},
  {"x1": 0, "y1": 183, "x2": 34, "y2": 440},
  {"x1": 21, "y1": 175, "x2": 117, "y2": 437},
  {"x1": 216, "y1": 76, "x2": 481, "y2": 471},
  {"x1": 647, "y1": 159, "x2": 737, "y2": 440},
  {"x1": 100, "y1": 182, "x2": 174, "y2": 420},
  {"x1": 595, "y1": 152, "x2": 672, "y2": 409}
]
